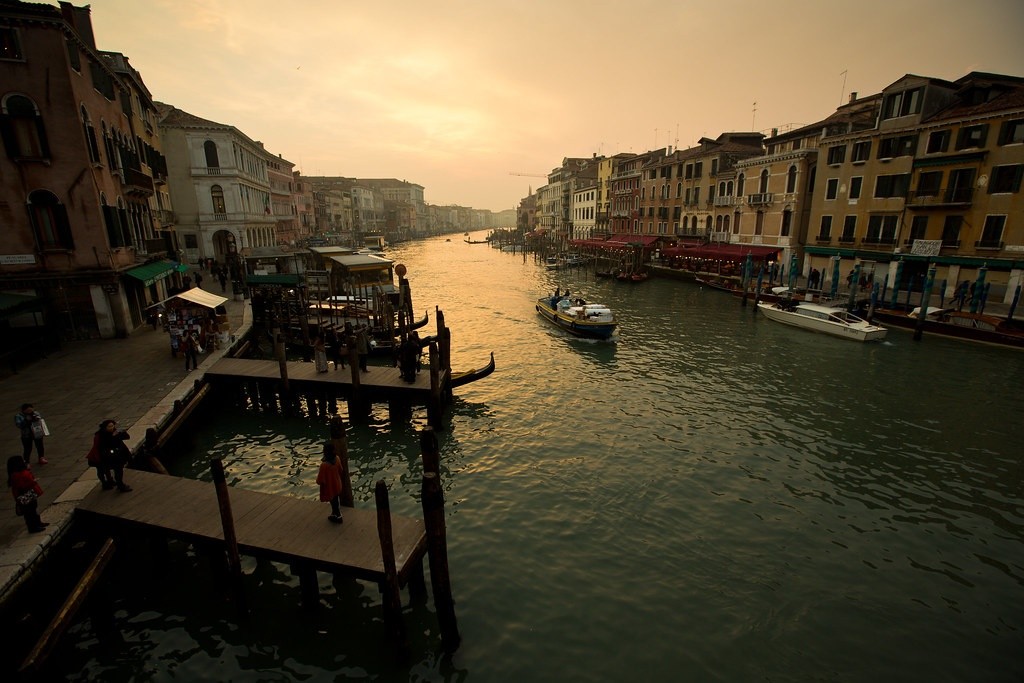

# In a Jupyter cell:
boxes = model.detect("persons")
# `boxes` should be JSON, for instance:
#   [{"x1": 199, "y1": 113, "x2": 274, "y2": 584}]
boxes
[
  {"x1": 181, "y1": 325, "x2": 199, "y2": 372},
  {"x1": 809, "y1": 269, "x2": 820, "y2": 289},
  {"x1": 146, "y1": 301, "x2": 160, "y2": 330},
  {"x1": 87, "y1": 421, "x2": 132, "y2": 492},
  {"x1": 182, "y1": 273, "x2": 192, "y2": 291},
  {"x1": 847, "y1": 267, "x2": 876, "y2": 294},
  {"x1": 197, "y1": 256, "x2": 228, "y2": 292},
  {"x1": 408, "y1": 329, "x2": 422, "y2": 375},
  {"x1": 14, "y1": 403, "x2": 48, "y2": 465},
  {"x1": 555, "y1": 288, "x2": 559, "y2": 296},
  {"x1": 949, "y1": 280, "x2": 970, "y2": 313},
  {"x1": 193, "y1": 271, "x2": 203, "y2": 290},
  {"x1": 314, "y1": 331, "x2": 328, "y2": 373},
  {"x1": 397, "y1": 340, "x2": 419, "y2": 385},
  {"x1": 965, "y1": 279, "x2": 979, "y2": 308},
  {"x1": 564, "y1": 289, "x2": 571, "y2": 296},
  {"x1": 284, "y1": 237, "x2": 365, "y2": 249},
  {"x1": 8, "y1": 455, "x2": 50, "y2": 534},
  {"x1": 316, "y1": 441, "x2": 347, "y2": 524},
  {"x1": 330, "y1": 329, "x2": 346, "y2": 370},
  {"x1": 353, "y1": 325, "x2": 372, "y2": 372}
]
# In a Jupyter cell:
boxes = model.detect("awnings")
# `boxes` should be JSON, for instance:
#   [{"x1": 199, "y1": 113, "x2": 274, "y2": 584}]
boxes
[
  {"x1": 127, "y1": 259, "x2": 190, "y2": 288},
  {"x1": 527, "y1": 230, "x2": 546, "y2": 238}
]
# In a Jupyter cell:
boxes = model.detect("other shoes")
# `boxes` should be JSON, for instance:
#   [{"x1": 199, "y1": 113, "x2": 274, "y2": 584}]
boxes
[
  {"x1": 39, "y1": 522, "x2": 49, "y2": 527},
  {"x1": 328, "y1": 515, "x2": 337, "y2": 523},
  {"x1": 41, "y1": 527, "x2": 46, "y2": 531},
  {"x1": 40, "y1": 457, "x2": 48, "y2": 465},
  {"x1": 102, "y1": 484, "x2": 113, "y2": 491},
  {"x1": 336, "y1": 517, "x2": 343, "y2": 524},
  {"x1": 27, "y1": 463, "x2": 32, "y2": 469},
  {"x1": 188, "y1": 369, "x2": 192, "y2": 372},
  {"x1": 119, "y1": 487, "x2": 130, "y2": 492},
  {"x1": 107, "y1": 480, "x2": 116, "y2": 486},
  {"x1": 117, "y1": 487, "x2": 119, "y2": 490}
]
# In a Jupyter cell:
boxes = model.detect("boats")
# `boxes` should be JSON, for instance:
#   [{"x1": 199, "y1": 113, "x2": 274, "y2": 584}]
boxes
[
  {"x1": 545, "y1": 259, "x2": 569, "y2": 269},
  {"x1": 695, "y1": 277, "x2": 827, "y2": 304},
  {"x1": 243, "y1": 235, "x2": 495, "y2": 398},
  {"x1": 596, "y1": 267, "x2": 648, "y2": 283},
  {"x1": 863, "y1": 304, "x2": 1024, "y2": 349},
  {"x1": 536, "y1": 294, "x2": 620, "y2": 339},
  {"x1": 464, "y1": 240, "x2": 488, "y2": 244},
  {"x1": 757, "y1": 304, "x2": 887, "y2": 341},
  {"x1": 547, "y1": 253, "x2": 587, "y2": 266}
]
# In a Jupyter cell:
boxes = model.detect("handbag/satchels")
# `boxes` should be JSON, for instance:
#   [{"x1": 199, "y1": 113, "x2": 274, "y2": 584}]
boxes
[
  {"x1": 17, "y1": 489, "x2": 39, "y2": 505},
  {"x1": 16, "y1": 505, "x2": 23, "y2": 516},
  {"x1": 31, "y1": 420, "x2": 45, "y2": 438}
]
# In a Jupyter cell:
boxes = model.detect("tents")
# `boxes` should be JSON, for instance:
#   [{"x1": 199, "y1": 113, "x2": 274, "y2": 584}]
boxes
[
  {"x1": 661, "y1": 242, "x2": 784, "y2": 262},
  {"x1": 570, "y1": 233, "x2": 659, "y2": 250}
]
